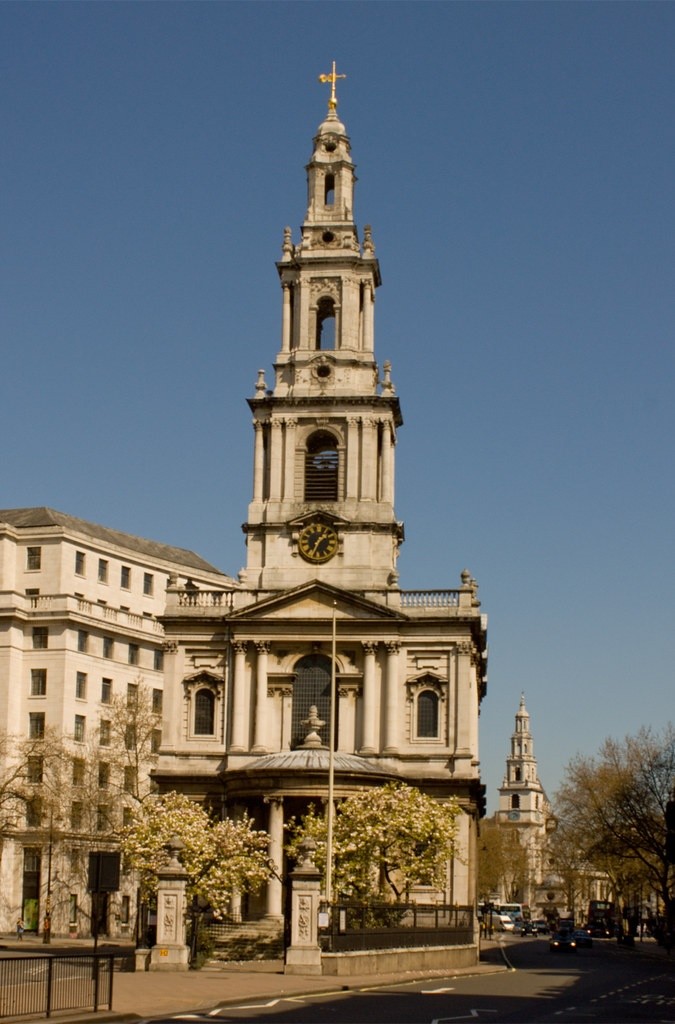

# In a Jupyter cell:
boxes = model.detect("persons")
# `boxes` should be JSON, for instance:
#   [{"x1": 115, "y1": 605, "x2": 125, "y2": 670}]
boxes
[{"x1": 15, "y1": 919, "x2": 25, "y2": 941}]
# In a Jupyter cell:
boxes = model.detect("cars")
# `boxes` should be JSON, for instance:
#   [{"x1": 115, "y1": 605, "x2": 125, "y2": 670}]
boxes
[
  {"x1": 550, "y1": 927, "x2": 593, "y2": 955},
  {"x1": 514, "y1": 922, "x2": 538, "y2": 937}
]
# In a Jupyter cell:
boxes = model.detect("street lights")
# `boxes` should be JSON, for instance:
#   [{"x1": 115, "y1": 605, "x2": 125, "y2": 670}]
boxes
[{"x1": 42, "y1": 800, "x2": 64, "y2": 943}]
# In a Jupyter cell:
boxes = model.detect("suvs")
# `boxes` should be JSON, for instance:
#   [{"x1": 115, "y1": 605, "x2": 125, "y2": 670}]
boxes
[{"x1": 527, "y1": 919, "x2": 549, "y2": 933}]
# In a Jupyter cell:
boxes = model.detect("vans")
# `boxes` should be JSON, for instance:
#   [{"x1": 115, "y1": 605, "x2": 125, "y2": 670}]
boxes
[{"x1": 491, "y1": 915, "x2": 515, "y2": 931}]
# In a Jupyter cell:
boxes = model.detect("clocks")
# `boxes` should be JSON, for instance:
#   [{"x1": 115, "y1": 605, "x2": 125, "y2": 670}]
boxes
[{"x1": 299, "y1": 522, "x2": 341, "y2": 564}]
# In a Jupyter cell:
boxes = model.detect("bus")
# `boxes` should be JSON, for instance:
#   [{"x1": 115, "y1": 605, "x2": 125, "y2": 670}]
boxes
[
  {"x1": 478, "y1": 903, "x2": 531, "y2": 924},
  {"x1": 586, "y1": 900, "x2": 616, "y2": 929}
]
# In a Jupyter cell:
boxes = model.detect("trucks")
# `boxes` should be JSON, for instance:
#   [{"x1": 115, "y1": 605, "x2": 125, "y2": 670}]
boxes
[{"x1": 555, "y1": 914, "x2": 574, "y2": 932}]
